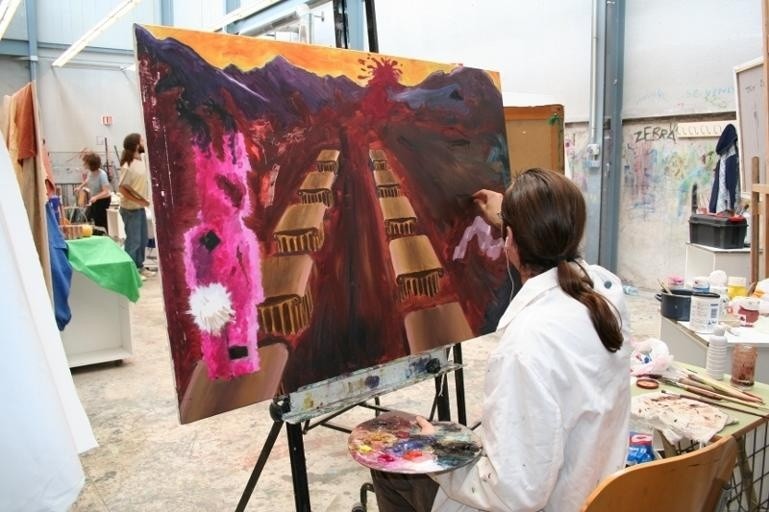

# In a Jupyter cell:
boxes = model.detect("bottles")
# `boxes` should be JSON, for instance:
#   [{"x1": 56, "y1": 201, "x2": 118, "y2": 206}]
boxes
[
  {"x1": 730, "y1": 344, "x2": 758, "y2": 390},
  {"x1": 738, "y1": 297, "x2": 762, "y2": 328},
  {"x1": 706, "y1": 324, "x2": 727, "y2": 380}
]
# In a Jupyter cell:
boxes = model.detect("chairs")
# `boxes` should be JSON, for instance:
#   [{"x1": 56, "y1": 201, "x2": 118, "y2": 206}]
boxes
[
  {"x1": 63, "y1": 187, "x2": 92, "y2": 226},
  {"x1": 578, "y1": 434, "x2": 737, "y2": 512}
]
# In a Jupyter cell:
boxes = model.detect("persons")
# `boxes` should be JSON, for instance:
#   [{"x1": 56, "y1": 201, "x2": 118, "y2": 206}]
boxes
[
  {"x1": 73, "y1": 153, "x2": 113, "y2": 233},
  {"x1": 119, "y1": 133, "x2": 158, "y2": 282},
  {"x1": 368, "y1": 166, "x2": 636, "y2": 512}
]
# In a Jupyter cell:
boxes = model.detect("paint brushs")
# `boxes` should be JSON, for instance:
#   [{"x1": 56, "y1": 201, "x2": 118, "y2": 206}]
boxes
[
  {"x1": 650, "y1": 366, "x2": 769, "y2": 418},
  {"x1": 725, "y1": 200, "x2": 749, "y2": 217}
]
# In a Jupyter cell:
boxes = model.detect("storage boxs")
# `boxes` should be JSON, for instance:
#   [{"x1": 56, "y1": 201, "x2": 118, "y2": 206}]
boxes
[{"x1": 689, "y1": 214, "x2": 747, "y2": 248}]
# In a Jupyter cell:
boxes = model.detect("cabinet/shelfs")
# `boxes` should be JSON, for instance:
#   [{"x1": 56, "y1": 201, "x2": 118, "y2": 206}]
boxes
[
  {"x1": 60, "y1": 235, "x2": 135, "y2": 368},
  {"x1": 622, "y1": 242, "x2": 769, "y2": 512}
]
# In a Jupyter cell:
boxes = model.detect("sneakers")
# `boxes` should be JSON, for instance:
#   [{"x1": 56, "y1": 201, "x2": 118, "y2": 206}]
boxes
[
  {"x1": 140, "y1": 276, "x2": 146, "y2": 281},
  {"x1": 141, "y1": 270, "x2": 156, "y2": 277}
]
